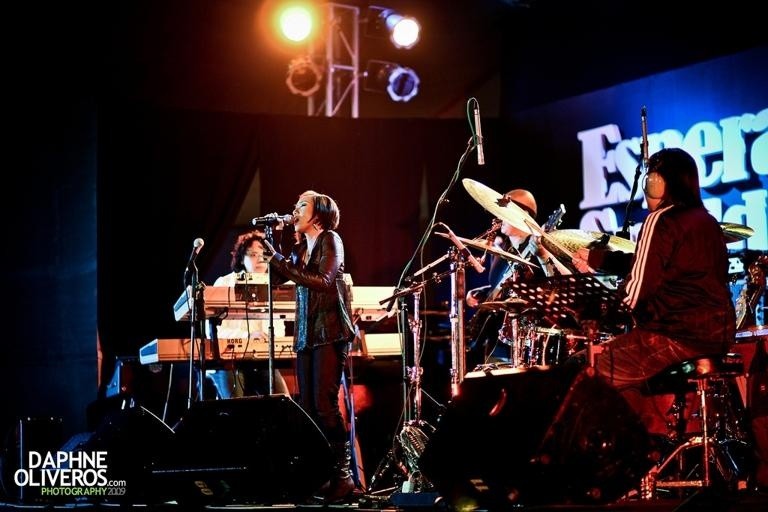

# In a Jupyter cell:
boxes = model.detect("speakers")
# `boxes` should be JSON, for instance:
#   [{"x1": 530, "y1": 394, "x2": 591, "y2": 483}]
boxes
[
  {"x1": 47, "y1": 405, "x2": 177, "y2": 504},
  {"x1": 170, "y1": 394, "x2": 337, "y2": 506},
  {"x1": 416, "y1": 396, "x2": 599, "y2": 512}
]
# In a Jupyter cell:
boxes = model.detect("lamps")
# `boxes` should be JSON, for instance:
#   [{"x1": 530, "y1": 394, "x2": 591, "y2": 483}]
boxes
[
  {"x1": 283, "y1": 53, "x2": 325, "y2": 97},
  {"x1": 360, "y1": 9, "x2": 421, "y2": 102}
]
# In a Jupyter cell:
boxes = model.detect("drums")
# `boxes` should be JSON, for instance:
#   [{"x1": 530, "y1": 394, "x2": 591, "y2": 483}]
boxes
[
  {"x1": 729, "y1": 329, "x2": 768, "y2": 391},
  {"x1": 518, "y1": 327, "x2": 613, "y2": 366}
]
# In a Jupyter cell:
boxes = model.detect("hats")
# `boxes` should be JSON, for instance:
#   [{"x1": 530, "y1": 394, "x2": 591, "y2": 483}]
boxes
[{"x1": 504, "y1": 189, "x2": 537, "y2": 216}]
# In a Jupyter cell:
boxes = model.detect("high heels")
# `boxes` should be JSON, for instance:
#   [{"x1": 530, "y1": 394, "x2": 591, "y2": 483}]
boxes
[{"x1": 323, "y1": 477, "x2": 355, "y2": 506}]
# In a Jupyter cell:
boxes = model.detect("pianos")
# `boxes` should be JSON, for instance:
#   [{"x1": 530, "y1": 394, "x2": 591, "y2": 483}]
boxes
[
  {"x1": 139, "y1": 332, "x2": 402, "y2": 365},
  {"x1": 173, "y1": 285, "x2": 398, "y2": 322}
]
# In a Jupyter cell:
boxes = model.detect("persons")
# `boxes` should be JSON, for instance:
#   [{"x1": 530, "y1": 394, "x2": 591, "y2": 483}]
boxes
[
  {"x1": 465, "y1": 190, "x2": 538, "y2": 363},
  {"x1": 262, "y1": 190, "x2": 364, "y2": 505},
  {"x1": 533, "y1": 148, "x2": 736, "y2": 475},
  {"x1": 203, "y1": 231, "x2": 291, "y2": 402}
]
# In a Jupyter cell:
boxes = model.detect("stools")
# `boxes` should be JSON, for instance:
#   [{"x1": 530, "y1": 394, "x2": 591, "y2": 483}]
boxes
[{"x1": 634, "y1": 351, "x2": 751, "y2": 491}]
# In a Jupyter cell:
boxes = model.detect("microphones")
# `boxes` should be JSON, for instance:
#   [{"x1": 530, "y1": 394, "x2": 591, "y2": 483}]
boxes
[
  {"x1": 642, "y1": 116, "x2": 649, "y2": 168},
  {"x1": 185, "y1": 238, "x2": 204, "y2": 272},
  {"x1": 474, "y1": 109, "x2": 485, "y2": 165},
  {"x1": 252, "y1": 214, "x2": 294, "y2": 226},
  {"x1": 449, "y1": 231, "x2": 486, "y2": 274}
]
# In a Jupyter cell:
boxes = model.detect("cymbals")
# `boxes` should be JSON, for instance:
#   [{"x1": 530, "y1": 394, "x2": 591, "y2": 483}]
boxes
[
  {"x1": 541, "y1": 229, "x2": 637, "y2": 253},
  {"x1": 719, "y1": 223, "x2": 755, "y2": 240},
  {"x1": 462, "y1": 178, "x2": 541, "y2": 237},
  {"x1": 433, "y1": 231, "x2": 540, "y2": 269}
]
